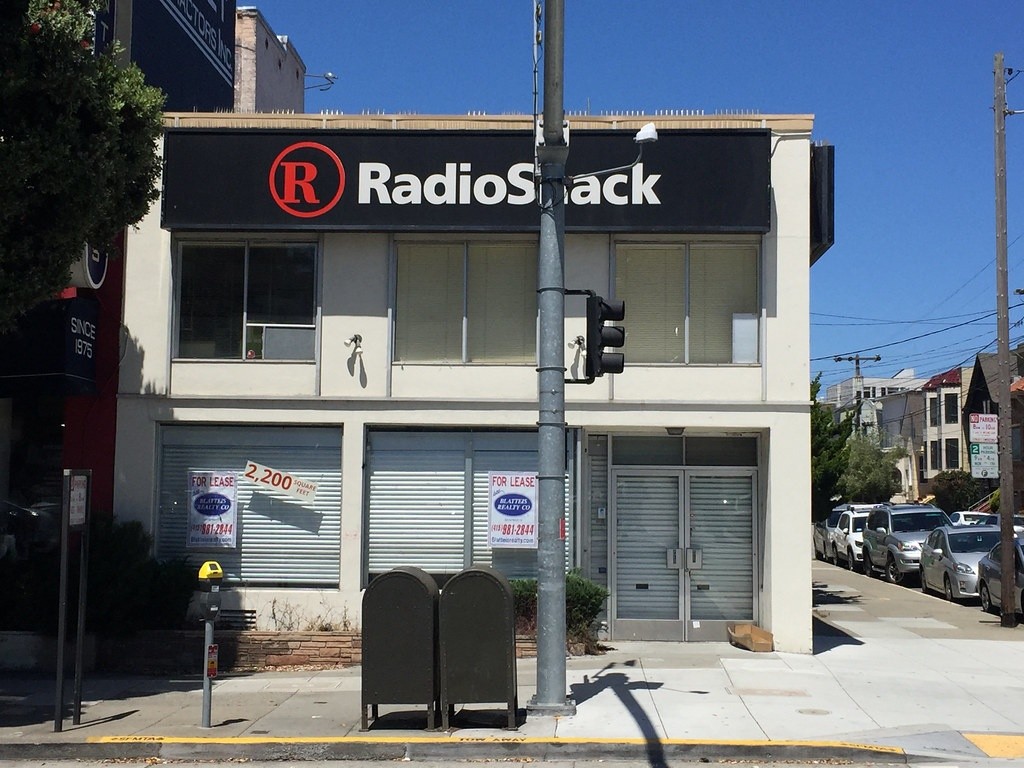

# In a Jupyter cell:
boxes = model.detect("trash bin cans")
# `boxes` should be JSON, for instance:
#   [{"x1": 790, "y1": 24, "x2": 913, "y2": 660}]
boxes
[{"x1": 360, "y1": 564, "x2": 518, "y2": 730}]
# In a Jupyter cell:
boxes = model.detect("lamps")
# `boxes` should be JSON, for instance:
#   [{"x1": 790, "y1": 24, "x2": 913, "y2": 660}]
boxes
[
  {"x1": 664, "y1": 427, "x2": 686, "y2": 435},
  {"x1": 567, "y1": 335, "x2": 587, "y2": 359},
  {"x1": 343, "y1": 333, "x2": 363, "y2": 355},
  {"x1": 634, "y1": 121, "x2": 659, "y2": 144}
]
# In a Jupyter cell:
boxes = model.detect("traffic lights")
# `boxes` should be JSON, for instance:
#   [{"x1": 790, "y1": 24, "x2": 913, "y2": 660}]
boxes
[{"x1": 585, "y1": 296, "x2": 626, "y2": 377}]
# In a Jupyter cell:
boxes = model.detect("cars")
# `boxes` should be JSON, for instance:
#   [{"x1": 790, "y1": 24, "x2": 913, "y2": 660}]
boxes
[
  {"x1": 0, "y1": 498, "x2": 63, "y2": 535},
  {"x1": 919, "y1": 525, "x2": 1002, "y2": 602},
  {"x1": 948, "y1": 511, "x2": 990, "y2": 527},
  {"x1": 977, "y1": 538, "x2": 1024, "y2": 615}
]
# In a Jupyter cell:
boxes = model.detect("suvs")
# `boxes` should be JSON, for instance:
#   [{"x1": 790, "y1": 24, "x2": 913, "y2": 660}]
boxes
[
  {"x1": 862, "y1": 502, "x2": 956, "y2": 584},
  {"x1": 975, "y1": 514, "x2": 1024, "y2": 539},
  {"x1": 813, "y1": 509, "x2": 847, "y2": 562},
  {"x1": 831, "y1": 503, "x2": 882, "y2": 571}
]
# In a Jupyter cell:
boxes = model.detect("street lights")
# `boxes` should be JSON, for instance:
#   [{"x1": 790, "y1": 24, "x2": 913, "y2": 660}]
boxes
[{"x1": 526, "y1": 119, "x2": 658, "y2": 717}]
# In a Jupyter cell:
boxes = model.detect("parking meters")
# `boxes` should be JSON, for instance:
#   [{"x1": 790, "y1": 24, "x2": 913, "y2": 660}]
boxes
[{"x1": 197, "y1": 560, "x2": 223, "y2": 728}]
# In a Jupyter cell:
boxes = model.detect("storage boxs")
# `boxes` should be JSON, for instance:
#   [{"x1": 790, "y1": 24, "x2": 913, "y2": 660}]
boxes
[{"x1": 727, "y1": 624, "x2": 773, "y2": 652}]
